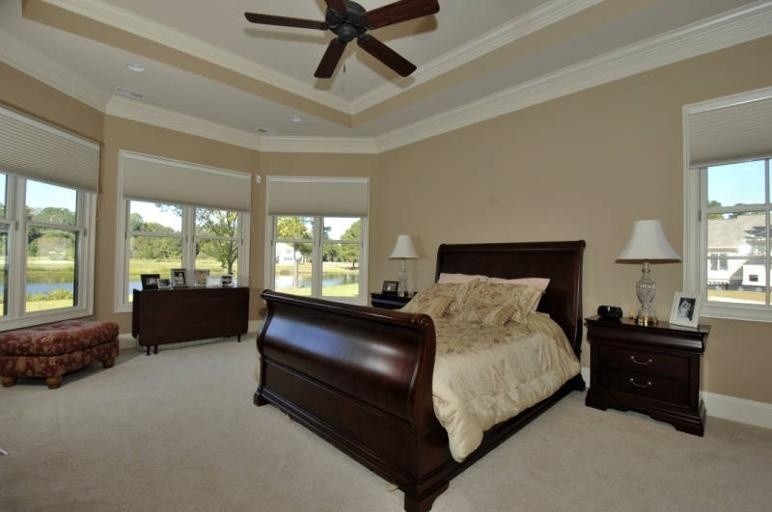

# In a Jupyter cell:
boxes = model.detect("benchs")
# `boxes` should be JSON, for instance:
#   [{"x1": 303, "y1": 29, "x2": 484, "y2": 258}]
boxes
[{"x1": 0, "y1": 320, "x2": 120, "y2": 390}]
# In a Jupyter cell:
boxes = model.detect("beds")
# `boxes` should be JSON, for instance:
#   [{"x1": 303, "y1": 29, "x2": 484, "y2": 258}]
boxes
[{"x1": 254, "y1": 241, "x2": 588, "y2": 512}]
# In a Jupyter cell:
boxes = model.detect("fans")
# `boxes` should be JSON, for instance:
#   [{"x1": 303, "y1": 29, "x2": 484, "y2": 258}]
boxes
[{"x1": 242, "y1": 0, "x2": 440, "y2": 80}]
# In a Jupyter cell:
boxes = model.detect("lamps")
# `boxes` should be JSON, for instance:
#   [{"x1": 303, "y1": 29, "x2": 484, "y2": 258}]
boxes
[
  {"x1": 388, "y1": 235, "x2": 419, "y2": 297},
  {"x1": 615, "y1": 220, "x2": 683, "y2": 326}
]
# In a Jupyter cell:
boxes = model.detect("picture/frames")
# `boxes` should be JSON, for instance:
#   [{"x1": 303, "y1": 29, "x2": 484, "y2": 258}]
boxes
[
  {"x1": 157, "y1": 278, "x2": 173, "y2": 290},
  {"x1": 382, "y1": 279, "x2": 399, "y2": 292},
  {"x1": 668, "y1": 293, "x2": 702, "y2": 329},
  {"x1": 140, "y1": 274, "x2": 159, "y2": 288},
  {"x1": 170, "y1": 268, "x2": 187, "y2": 287}
]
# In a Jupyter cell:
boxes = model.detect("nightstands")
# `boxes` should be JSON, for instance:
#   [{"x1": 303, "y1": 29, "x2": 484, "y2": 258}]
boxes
[
  {"x1": 369, "y1": 292, "x2": 418, "y2": 310},
  {"x1": 582, "y1": 311, "x2": 711, "y2": 437}
]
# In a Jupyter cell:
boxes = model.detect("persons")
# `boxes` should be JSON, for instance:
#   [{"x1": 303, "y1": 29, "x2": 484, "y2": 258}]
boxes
[{"x1": 676, "y1": 297, "x2": 695, "y2": 324}]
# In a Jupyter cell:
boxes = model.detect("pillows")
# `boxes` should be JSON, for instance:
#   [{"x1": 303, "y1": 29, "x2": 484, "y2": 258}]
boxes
[{"x1": 398, "y1": 269, "x2": 552, "y2": 327}]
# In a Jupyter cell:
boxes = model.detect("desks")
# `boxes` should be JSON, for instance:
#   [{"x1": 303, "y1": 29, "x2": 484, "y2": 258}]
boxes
[{"x1": 132, "y1": 285, "x2": 249, "y2": 355}]
